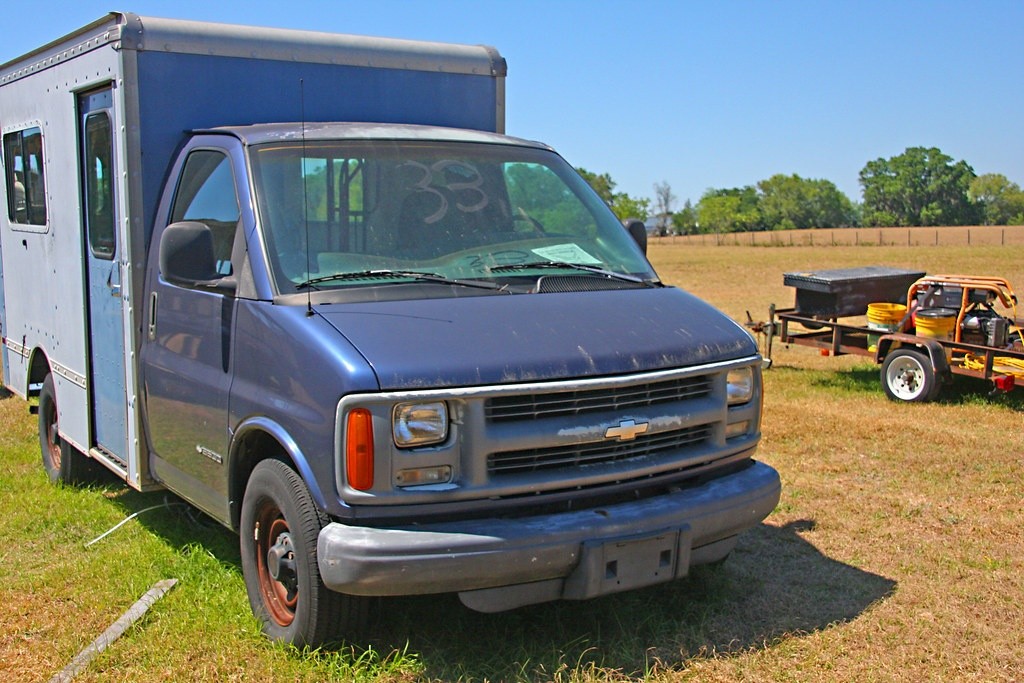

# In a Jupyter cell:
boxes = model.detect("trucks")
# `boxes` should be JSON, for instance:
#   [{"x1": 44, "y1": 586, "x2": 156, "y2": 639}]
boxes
[{"x1": 0, "y1": 10, "x2": 782, "y2": 652}]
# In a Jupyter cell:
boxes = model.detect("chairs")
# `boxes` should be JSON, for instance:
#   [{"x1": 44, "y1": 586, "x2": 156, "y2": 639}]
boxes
[{"x1": 394, "y1": 180, "x2": 500, "y2": 263}]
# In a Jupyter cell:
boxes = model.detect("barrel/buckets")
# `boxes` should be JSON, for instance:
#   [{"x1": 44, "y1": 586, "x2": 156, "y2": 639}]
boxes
[
  {"x1": 916, "y1": 307, "x2": 957, "y2": 364},
  {"x1": 867, "y1": 302, "x2": 907, "y2": 352}
]
